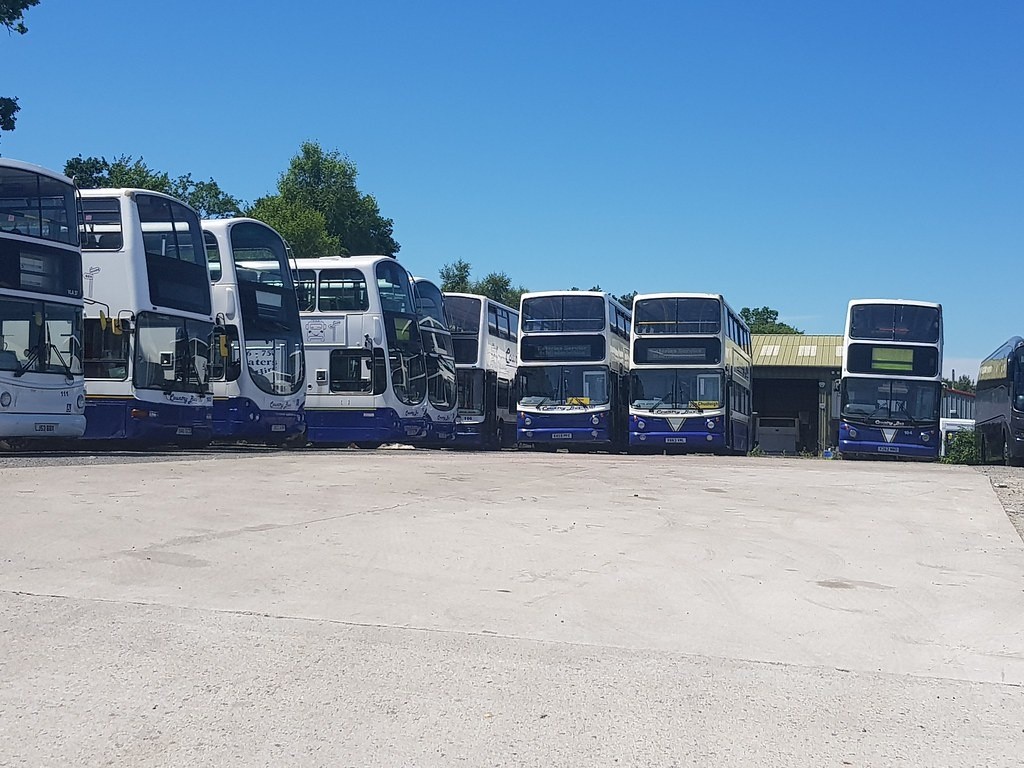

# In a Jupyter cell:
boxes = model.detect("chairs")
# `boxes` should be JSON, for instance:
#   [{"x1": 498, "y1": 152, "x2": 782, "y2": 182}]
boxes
[{"x1": 330, "y1": 296, "x2": 352, "y2": 309}]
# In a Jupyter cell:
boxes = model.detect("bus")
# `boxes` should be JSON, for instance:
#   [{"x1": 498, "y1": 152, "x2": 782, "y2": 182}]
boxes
[
  {"x1": 208, "y1": 254, "x2": 428, "y2": 450},
  {"x1": 515, "y1": 290, "x2": 632, "y2": 453},
  {"x1": 81, "y1": 217, "x2": 308, "y2": 443},
  {"x1": 74, "y1": 187, "x2": 229, "y2": 450},
  {"x1": 262, "y1": 277, "x2": 460, "y2": 446},
  {"x1": 628, "y1": 292, "x2": 753, "y2": 455},
  {"x1": 837, "y1": 298, "x2": 945, "y2": 462},
  {"x1": 444, "y1": 292, "x2": 551, "y2": 451},
  {"x1": 0, "y1": 156, "x2": 110, "y2": 452},
  {"x1": 974, "y1": 334, "x2": 1024, "y2": 467}
]
